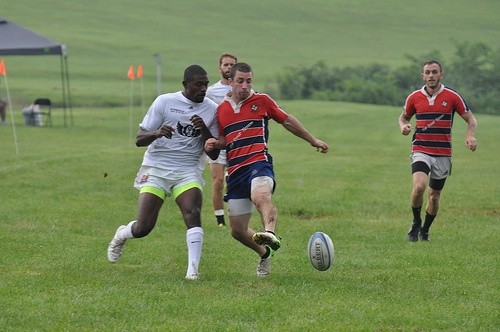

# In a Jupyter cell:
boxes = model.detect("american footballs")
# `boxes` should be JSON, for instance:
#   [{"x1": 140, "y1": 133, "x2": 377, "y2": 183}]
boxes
[{"x1": 307, "y1": 231, "x2": 335, "y2": 272}]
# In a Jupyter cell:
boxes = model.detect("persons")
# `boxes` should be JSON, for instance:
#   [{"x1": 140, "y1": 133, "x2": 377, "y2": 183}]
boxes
[
  {"x1": 207, "y1": 53, "x2": 236, "y2": 227},
  {"x1": 206, "y1": 61, "x2": 328, "y2": 275},
  {"x1": 399, "y1": 59, "x2": 476, "y2": 241},
  {"x1": 107, "y1": 66, "x2": 221, "y2": 280}
]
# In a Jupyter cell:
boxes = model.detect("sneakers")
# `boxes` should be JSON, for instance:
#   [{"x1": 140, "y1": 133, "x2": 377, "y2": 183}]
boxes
[
  {"x1": 257, "y1": 246, "x2": 275, "y2": 276},
  {"x1": 107, "y1": 225, "x2": 127, "y2": 263},
  {"x1": 419, "y1": 226, "x2": 430, "y2": 241},
  {"x1": 408, "y1": 218, "x2": 422, "y2": 242},
  {"x1": 252, "y1": 232, "x2": 281, "y2": 250}
]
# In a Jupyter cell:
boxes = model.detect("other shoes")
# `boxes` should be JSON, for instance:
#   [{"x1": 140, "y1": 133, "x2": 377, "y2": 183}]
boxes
[
  {"x1": 216, "y1": 216, "x2": 225, "y2": 228},
  {"x1": 223, "y1": 193, "x2": 228, "y2": 202}
]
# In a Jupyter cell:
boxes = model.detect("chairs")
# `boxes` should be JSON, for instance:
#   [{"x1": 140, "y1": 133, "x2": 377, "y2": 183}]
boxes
[{"x1": 29, "y1": 97, "x2": 52, "y2": 128}]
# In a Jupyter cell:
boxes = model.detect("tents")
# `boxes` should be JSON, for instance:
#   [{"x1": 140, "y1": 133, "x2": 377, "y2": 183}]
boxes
[{"x1": 0, "y1": 19, "x2": 72, "y2": 127}]
